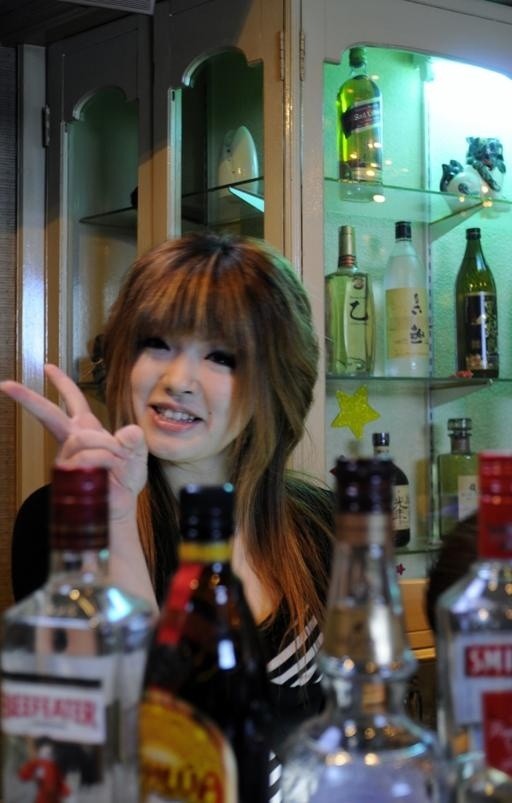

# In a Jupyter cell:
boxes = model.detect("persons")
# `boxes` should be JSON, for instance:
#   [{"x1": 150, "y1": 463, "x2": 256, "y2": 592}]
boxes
[{"x1": 0, "y1": 225, "x2": 335, "y2": 803}]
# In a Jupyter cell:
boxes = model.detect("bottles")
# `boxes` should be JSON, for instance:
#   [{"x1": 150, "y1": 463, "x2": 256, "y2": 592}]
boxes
[
  {"x1": 436, "y1": 416, "x2": 483, "y2": 543},
  {"x1": 324, "y1": 224, "x2": 378, "y2": 379},
  {"x1": 336, "y1": 45, "x2": 384, "y2": 204},
  {"x1": 379, "y1": 219, "x2": 432, "y2": 381},
  {"x1": 278, "y1": 450, "x2": 457, "y2": 803},
  {"x1": 452, "y1": 225, "x2": 502, "y2": 379},
  {"x1": 1, "y1": 461, "x2": 154, "y2": 803},
  {"x1": 425, "y1": 448, "x2": 511, "y2": 803},
  {"x1": 371, "y1": 431, "x2": 413, "y2": 547},
  {"x1": 135, "y1": 479, "x2": 274, "y2": 803}
]
